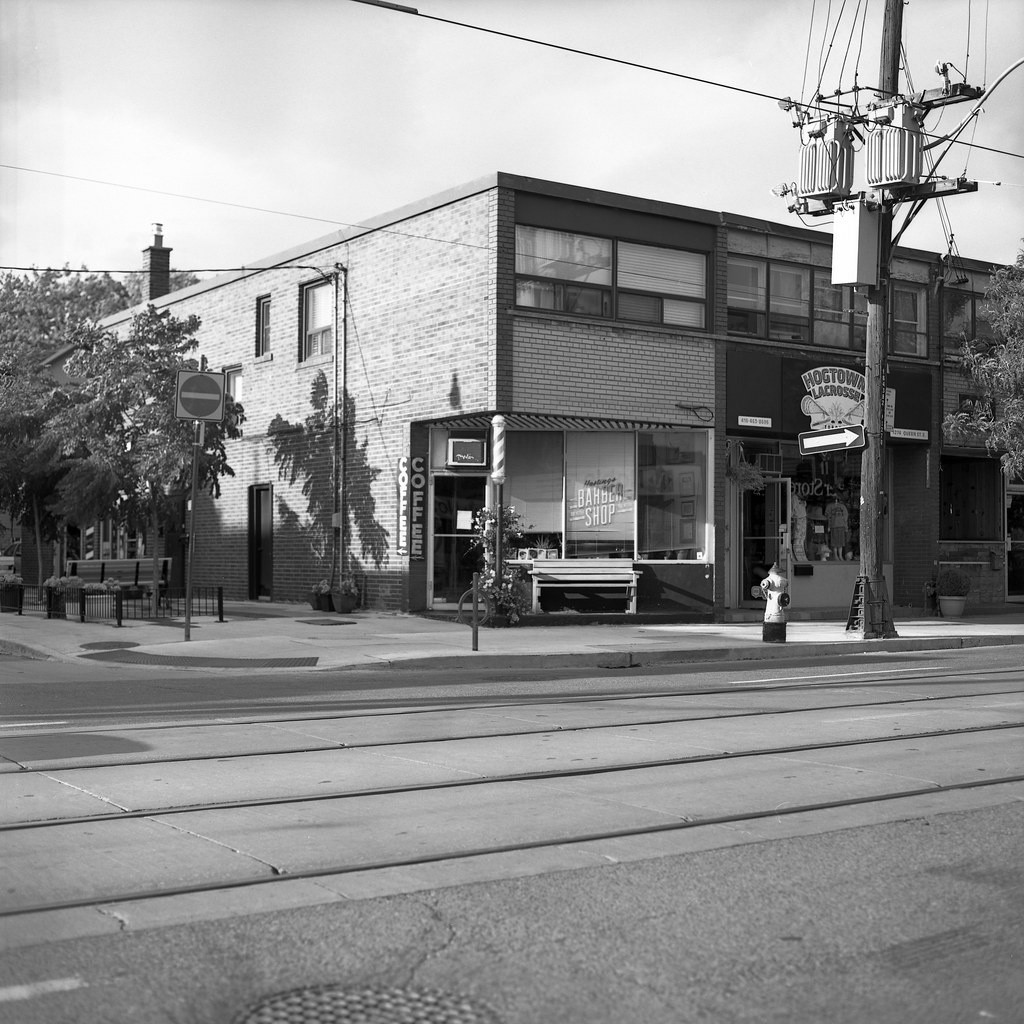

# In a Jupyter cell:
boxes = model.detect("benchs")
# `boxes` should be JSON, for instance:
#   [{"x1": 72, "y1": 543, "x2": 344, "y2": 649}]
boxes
[
  {"x1": 527, "y1": 558, "x2": 643, "y2": 614},
  {"x1": 66, "y1": 558, "x2": 173, "y2": 593}
]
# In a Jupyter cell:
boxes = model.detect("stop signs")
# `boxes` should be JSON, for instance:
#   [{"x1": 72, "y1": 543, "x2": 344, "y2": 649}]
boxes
[{"x1": 175, "y1": 370, "x2": 226, "y2": 423}]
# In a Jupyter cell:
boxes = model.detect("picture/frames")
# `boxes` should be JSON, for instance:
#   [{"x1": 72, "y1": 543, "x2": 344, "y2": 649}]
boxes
[
  {"x1": 547, "y1": 549, "x2": 558, "y2": 559},
  {"x1": 518, "y1": 548, "x2": 530, "y2": 560},
  {"x1": 679, "y1": 471, "x2": 695, "y2": 498},
  {"x1": 679, "y1": 518, "x2": 696, "y2": 544},
  {"x1": 681, "y1": 500, "x2": 695, "y2": 516}
]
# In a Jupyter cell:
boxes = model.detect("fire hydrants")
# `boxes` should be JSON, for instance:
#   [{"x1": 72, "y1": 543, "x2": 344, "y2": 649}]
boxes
[{"x1": 761, "y1": 561, "x2": 791, "y2": 640}]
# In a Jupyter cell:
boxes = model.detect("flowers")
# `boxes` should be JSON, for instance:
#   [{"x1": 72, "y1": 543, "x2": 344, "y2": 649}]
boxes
[{"x1": 464, "y1": 504, "x2": 529, "y2": 625}]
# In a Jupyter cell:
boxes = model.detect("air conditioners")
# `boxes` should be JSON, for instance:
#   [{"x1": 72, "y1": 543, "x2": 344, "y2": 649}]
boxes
[{"x1": 448, "y1": 437, "x2": 487, "y2": 467}]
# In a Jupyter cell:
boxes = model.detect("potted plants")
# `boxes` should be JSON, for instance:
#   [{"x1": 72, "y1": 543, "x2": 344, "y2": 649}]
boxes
[
  {"x1": 0, "y1": 572, "x2": 25, "y2": 612},
  {"x1": 308, "y1": 578, "x2": 360, "y2": 614},
  {"x1": 85, "y1": 580, "x2": 120, "y2": 619},
  {"x1": 936, "y1": 568, "x2": 971, "y2": 619},
  {"x1": 42, "y1": 575, "x2": 84, "y2": 603}
]
